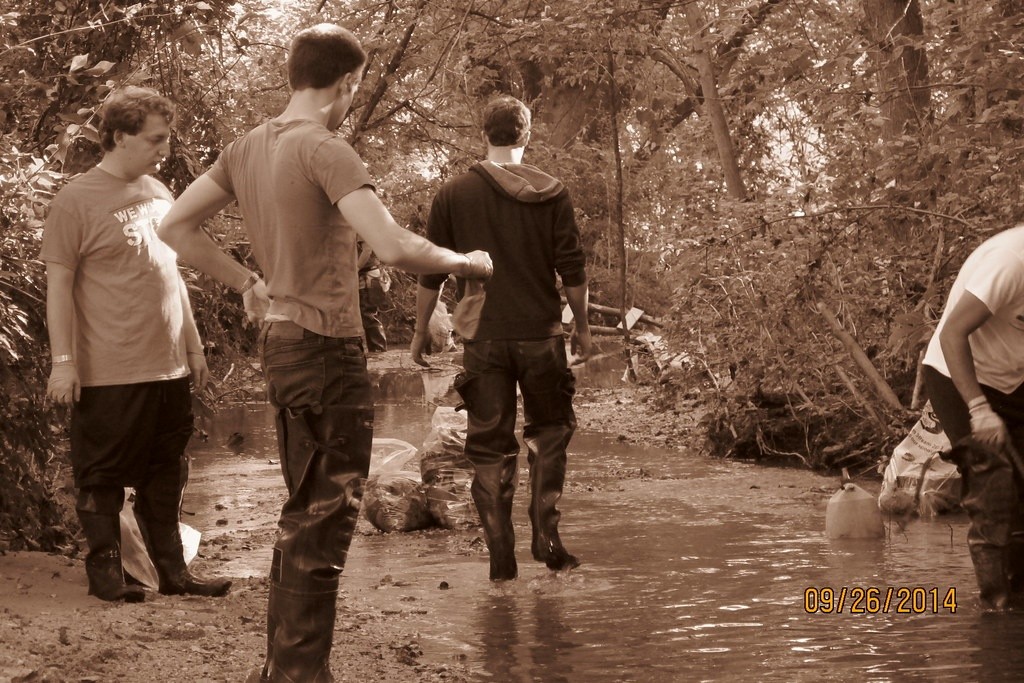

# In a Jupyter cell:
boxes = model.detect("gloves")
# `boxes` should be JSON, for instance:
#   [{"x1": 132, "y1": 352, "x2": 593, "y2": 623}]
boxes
[
  {"x1": 450, "y1": 276, "x2": 486, "y2": 340},
  {"x1": 188, "y1": 354, "x2": 210, "y2": 391},
  {"x1": 967, "y1": 403, "x2": 1006, "y2": 448},
  {"x1": 46, "y1": 364, "x2": 81, "y2": 410}
]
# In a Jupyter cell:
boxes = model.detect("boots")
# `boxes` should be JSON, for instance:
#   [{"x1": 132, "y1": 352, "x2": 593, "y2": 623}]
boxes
[
  {"x1": 78, "y1": 511, "x2": 146, "y2": 603},
  {"x1": 937, "y1": 427, "x2": 1024, "y2": 614},
  {"x1": 258, "y1": 407, "x2": 377, "y2": 683},
  {"x1": 455, "y1": 371, "x2": 521, "y2": 583},
  {"x1": 513, "y1": 368, "x2": 582, "y2": 570},
  {"x1": 133, "y1": 500, "x2": 233, "y2": 597},
  {"x1": 359, "y1": 288, "x2": 388, "y2": 353}
]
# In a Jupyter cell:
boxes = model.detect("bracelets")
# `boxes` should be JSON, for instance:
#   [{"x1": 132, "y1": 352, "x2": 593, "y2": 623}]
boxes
[
  {"x1": 238, "y1": 272, "x2": 259, "y2": 295},
  {"x1": 967, "y1": 395, "x2": 987, "y2": 410},
  {"x1": 52, "y1": 355, "x2": 72, "y2": 363}
]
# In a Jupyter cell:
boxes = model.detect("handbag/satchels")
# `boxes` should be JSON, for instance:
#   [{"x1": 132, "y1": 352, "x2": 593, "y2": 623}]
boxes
[{"x1": 366, "y1": 267, "x2": 393, "y2": 292}]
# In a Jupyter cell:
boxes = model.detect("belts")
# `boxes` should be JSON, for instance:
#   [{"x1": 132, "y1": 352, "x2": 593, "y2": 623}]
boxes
[{"x1": 260, "y1": 321, "x2": 308, "y2": 336}]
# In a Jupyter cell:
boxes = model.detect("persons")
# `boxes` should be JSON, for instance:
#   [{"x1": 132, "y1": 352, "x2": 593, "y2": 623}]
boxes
[
  {"x1": 411, "y1": 96, "x2": 592, "y2": 581},
  {"x1": 157, "y1": 23, "x2": 493, "y2": 683},
  {"x1": 922, "y1": 225, "x2": 1024, "y2": 612},
  {"x1": 38, "y1": 86, "x2": 233, "y2": 601}
]
